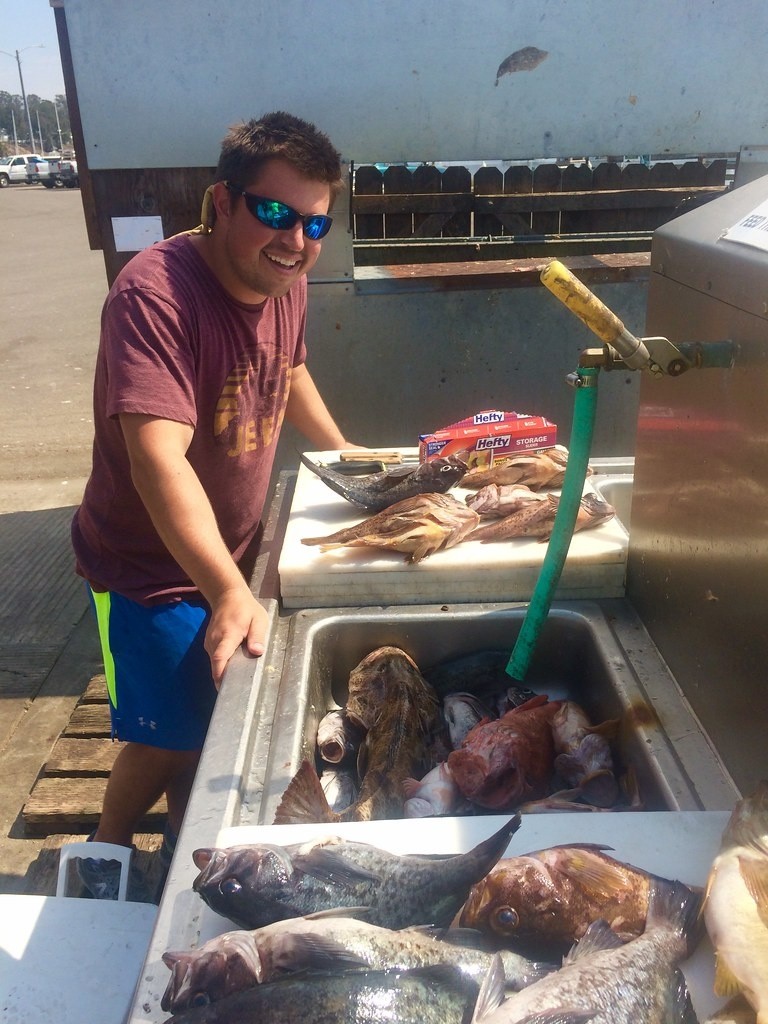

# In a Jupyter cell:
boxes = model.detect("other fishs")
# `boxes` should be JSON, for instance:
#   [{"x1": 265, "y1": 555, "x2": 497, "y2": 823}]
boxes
[
  {"x1": 299, "y1": 444, "x2": 616, "y2": 566},
  {"x1": 161, "y1": 645, "x2": 767, "y2": 1024}
]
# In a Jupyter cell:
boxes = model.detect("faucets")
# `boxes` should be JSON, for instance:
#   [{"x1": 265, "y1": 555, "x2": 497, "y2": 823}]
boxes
[{"x1": 504, "y1": 257, "x2": 738, "y2": 683}]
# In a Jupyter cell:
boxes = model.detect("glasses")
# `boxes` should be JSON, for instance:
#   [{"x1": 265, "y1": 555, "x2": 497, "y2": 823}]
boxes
[{"x1": 226, "y1": 184, "x2": 333, "y2": 240}]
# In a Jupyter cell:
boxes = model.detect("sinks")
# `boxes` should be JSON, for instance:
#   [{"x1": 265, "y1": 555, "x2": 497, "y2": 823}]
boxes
[{"x1": 228, "y1": 597, "x2": 752, "y2": 824}]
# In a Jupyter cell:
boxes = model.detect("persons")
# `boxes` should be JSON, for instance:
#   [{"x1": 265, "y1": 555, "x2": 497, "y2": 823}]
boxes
[{"x1": 71, "y1": 111, "x2": 368, "y2": 909}]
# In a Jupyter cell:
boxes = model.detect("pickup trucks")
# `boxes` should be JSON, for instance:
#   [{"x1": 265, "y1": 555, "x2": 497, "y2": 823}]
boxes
[
  {"x1": 26, "y1": 163, "x2": 64, "y2": 188},
  {"x1": 0, "y1": 154, "x2": 42, "y2": 188},
  {"x1": 57, "y1": 160, "x2": 80, "y2": 188}
]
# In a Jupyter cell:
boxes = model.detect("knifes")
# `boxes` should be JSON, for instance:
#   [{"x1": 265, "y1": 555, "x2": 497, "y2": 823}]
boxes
[{"x1": 318, "y1": 451, "x2": 403, "y2": 476}]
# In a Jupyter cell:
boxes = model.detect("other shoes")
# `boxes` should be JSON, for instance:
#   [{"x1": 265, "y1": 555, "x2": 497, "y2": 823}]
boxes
[
  {"x1": 75, "y1": 829, "x2": 151, "y2": 902},
  {"x1": 146, "y1": 851, "x2": 169, "y2": 906}
]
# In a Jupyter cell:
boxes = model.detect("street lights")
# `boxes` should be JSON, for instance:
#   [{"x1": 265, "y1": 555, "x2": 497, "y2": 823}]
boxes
[{"x1": 0, "y1": 45, "x2": 46, "y2": 154}]
